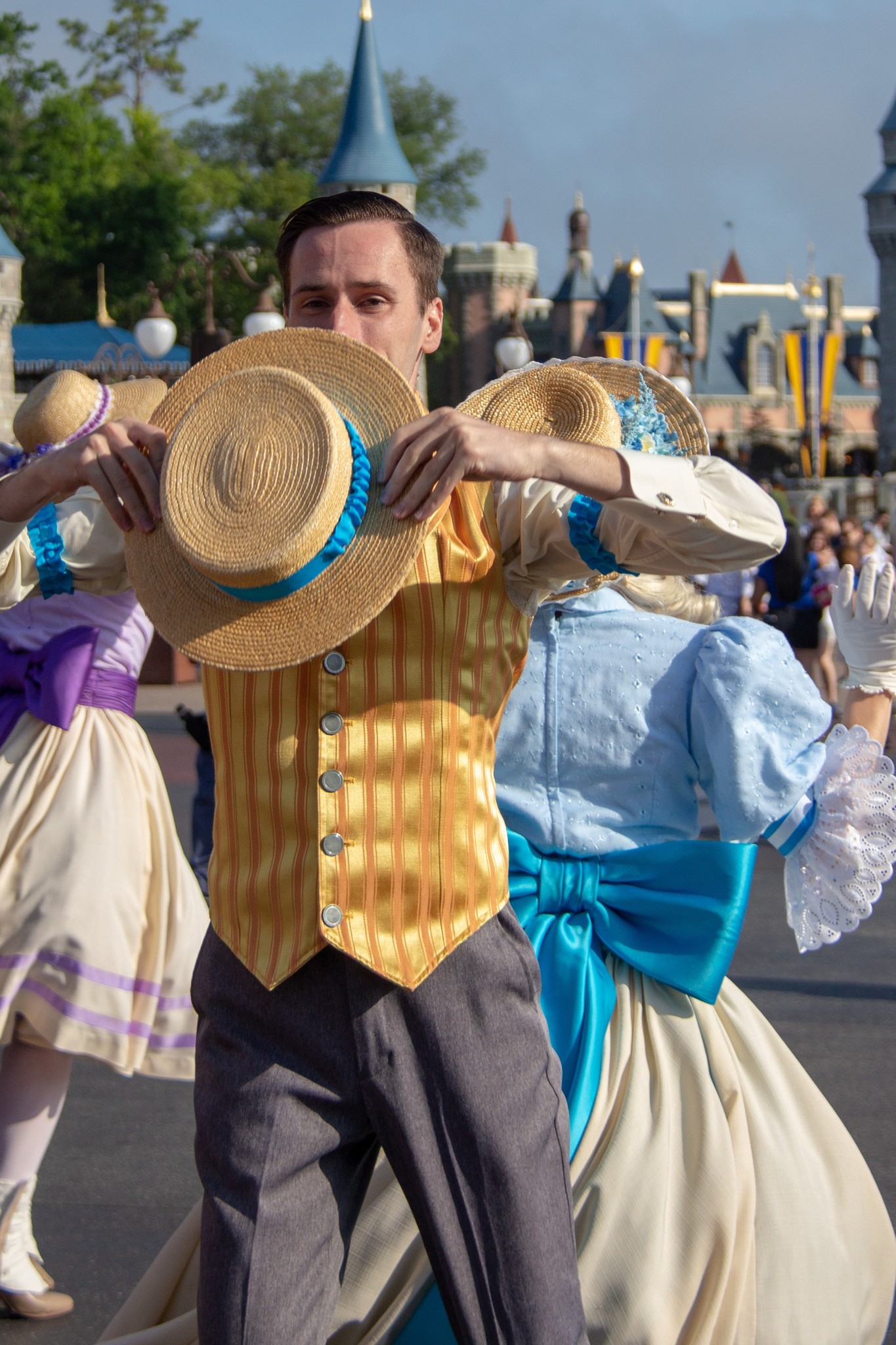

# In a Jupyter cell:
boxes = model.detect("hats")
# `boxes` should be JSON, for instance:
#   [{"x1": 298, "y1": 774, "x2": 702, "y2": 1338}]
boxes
[
  {"x1": 9, "y1": 368, "x2": 170, "y2": 480},
  {"x1": 460, "y1": 352, "x2": 710, "y2": 600},
  {"x1": 123, "y1": 327, "x2": 437, "y2": 674}
]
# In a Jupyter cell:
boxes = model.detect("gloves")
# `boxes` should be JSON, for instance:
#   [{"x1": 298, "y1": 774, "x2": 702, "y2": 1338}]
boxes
[{"x1": 828, "y1": 554, "x2": 896, "y2": 696}]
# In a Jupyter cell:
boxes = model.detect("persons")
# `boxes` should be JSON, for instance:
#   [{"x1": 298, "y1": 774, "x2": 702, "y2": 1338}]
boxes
[
  {"x1": 95, "y1": 355, "x2": 896, "y2": 1345},
  {"x1": 0, "y1": 192, "x2": 784, "y2": 1345},
  {"x1": 690, "y1": 435, "x2": 896, "y2": 724},
  {"x1": 0, "y1": 371, "x2": 210, "y2": 1325}
]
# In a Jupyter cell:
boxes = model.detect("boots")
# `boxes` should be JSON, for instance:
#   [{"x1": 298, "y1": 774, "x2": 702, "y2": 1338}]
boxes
[{"x1": 0, "y1": 1173, "x2": 77, "y2": 1321}]
